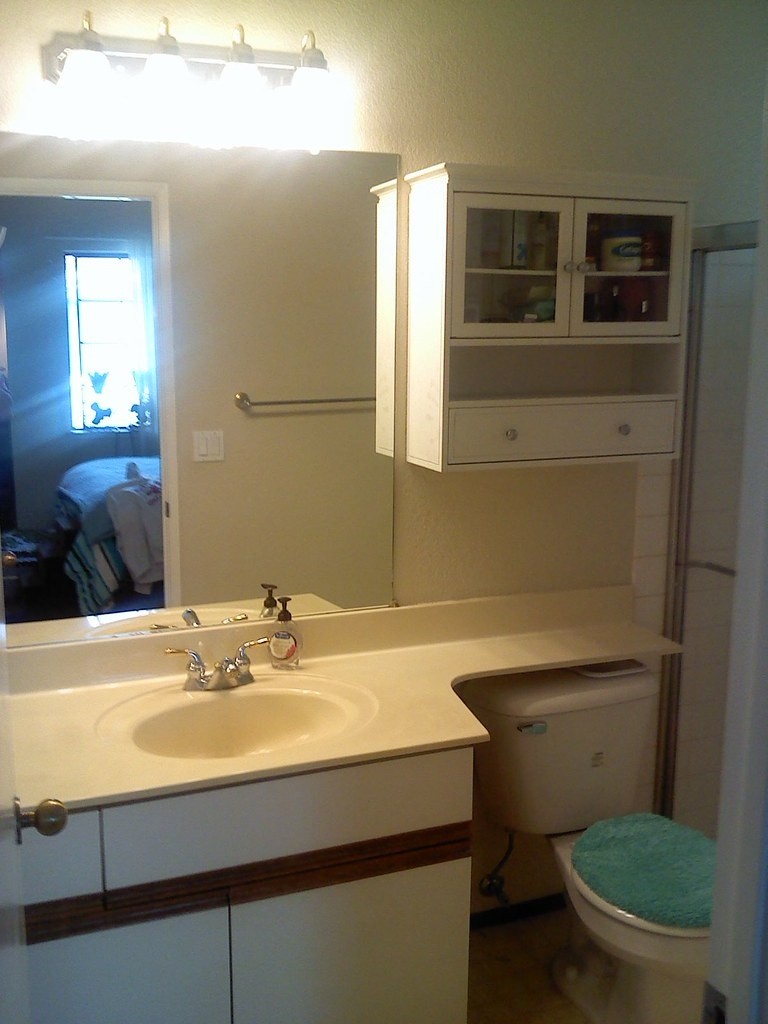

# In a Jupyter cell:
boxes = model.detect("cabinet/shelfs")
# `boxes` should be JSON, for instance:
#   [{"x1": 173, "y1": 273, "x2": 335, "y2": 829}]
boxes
[
  {"x1": 368, "y1": 178, "x2": 394, "y2": 458},
  {"x1": 403, "y1": 158, "x2": 700, "y2": 474},
  {"x1": 28, "y1": 749, "x2": 480, "y2": 1024}
]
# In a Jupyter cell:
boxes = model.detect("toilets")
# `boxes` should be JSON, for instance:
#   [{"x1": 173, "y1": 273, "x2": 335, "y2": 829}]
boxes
[{"x1": 462, "y1": 660, "x2": 718, "y2": 1024}]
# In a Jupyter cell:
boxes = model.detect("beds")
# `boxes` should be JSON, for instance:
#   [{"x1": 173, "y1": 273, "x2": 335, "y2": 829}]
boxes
[{"x1": 51, "y1": 454, "x2": 162, "y2": 610}]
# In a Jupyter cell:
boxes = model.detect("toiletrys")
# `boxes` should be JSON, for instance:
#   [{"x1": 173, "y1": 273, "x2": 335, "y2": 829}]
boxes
[
  {"x1": 259, "y1": 583, "x2": 281, "y2": 618},
  {"x1": 266, "y1": 596, "x2": 304, "y2": 670}
]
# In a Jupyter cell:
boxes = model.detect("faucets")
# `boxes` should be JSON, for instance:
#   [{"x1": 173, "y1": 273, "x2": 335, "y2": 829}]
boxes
[
  {"x1": 205, "y1": 655, "x2": 240, "y2": 691},
  {"x1": 182, "y1": 608, "x2": 201, "y2": 627}
]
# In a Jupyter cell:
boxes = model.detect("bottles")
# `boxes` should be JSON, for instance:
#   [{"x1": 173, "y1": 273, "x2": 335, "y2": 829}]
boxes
[{"x1": 527, "y1": 222, "x2": 553, "y2": 270}]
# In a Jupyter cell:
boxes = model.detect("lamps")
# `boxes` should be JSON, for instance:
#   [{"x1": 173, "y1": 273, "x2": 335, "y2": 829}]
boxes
[{"x1": 47, "y1": 8, "x2": 357, "y2": 135}]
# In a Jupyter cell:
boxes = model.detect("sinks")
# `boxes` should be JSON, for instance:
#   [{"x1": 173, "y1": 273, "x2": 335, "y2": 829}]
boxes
[
  {"x1": 84, "y1": 607, "x2": 261, "y2": 639},
  {"x1": 90, "y1": 671, "x2": 382, "y2": 783}
]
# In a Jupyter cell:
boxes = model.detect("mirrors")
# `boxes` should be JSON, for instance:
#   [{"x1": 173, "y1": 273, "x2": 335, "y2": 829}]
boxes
[{"x1": 1, "y1": 126, "x2": 399, "y2": 652}]
